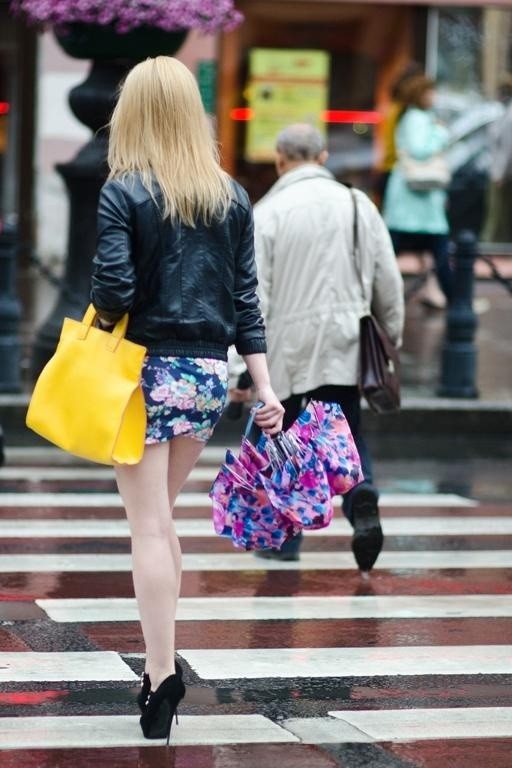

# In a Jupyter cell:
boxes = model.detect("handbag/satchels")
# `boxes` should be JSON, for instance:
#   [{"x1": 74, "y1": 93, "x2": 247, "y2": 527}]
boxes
[
  {"x1": 396, "y1": 140, "x2": 451, "y2": 192},
  {"x1": 358, "y1": 314, "x2": 400, "y2": 415},
  {"x1": 26, "y1": 301, "x2": 147, "y2": 466}
]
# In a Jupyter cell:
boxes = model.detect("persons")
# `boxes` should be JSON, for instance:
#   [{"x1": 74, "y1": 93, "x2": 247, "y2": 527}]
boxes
[
  {"x1": 227, "y1": 121, "x2": 406, "y2": 569},
  {"x1": 381, "y1": 74, "x2": 488, "y2": 314},
  {"x1": 88, "y1": 53, "x2": 286, "y2": 751}
]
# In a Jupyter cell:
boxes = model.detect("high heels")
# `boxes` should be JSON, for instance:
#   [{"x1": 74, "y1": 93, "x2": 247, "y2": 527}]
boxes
[{"x1": 136, "y1": 659, "x2": 186, "y2": 746}]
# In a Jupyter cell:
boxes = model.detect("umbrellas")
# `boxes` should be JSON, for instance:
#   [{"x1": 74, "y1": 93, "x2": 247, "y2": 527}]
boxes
[{"x1": 206, "y1": 399, "x2": 366, "y2": 554}]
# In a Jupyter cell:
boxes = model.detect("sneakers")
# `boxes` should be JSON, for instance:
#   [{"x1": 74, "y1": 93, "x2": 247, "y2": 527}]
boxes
[
  {"x1": 350, "y1": 486, "x2": 384, "y2": 571},
  {"x1": 255, "y1": 540, "x2": 299, "y2": 561}
]
